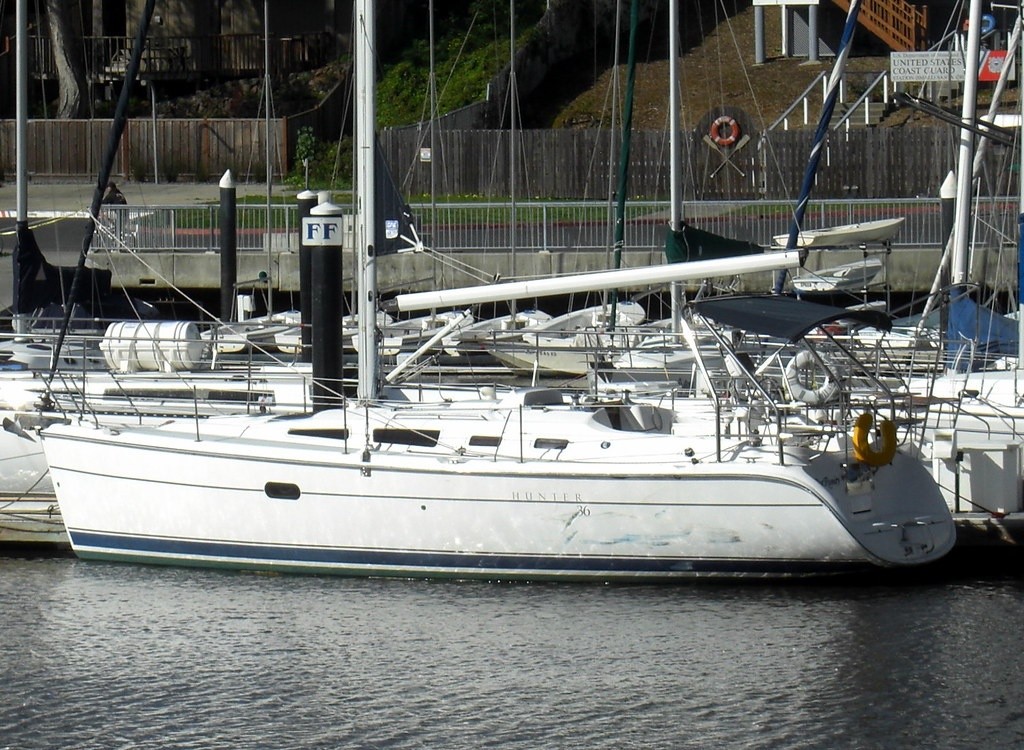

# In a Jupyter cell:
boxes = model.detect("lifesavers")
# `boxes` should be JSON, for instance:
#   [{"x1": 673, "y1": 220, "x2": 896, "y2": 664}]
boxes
[
  {"x1": 855, "y1": 414, "x2": 897, "y2": 468},
  {"x1": 786, "y1": 350, "x2": 839, "y2": 405},
  {"x1": 711, "y1": 117, "x2": 739, "y2": 145}
]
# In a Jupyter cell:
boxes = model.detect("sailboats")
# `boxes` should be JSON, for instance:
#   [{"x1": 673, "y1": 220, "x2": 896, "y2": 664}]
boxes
[{"x1": 1, "y1": 0, "x2": 1023, "y2": 584}]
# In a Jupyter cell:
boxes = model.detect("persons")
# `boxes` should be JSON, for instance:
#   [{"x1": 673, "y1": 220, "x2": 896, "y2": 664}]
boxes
[{"x1": 99, "y1": 181, "x2": 128, "y2": 230}]
